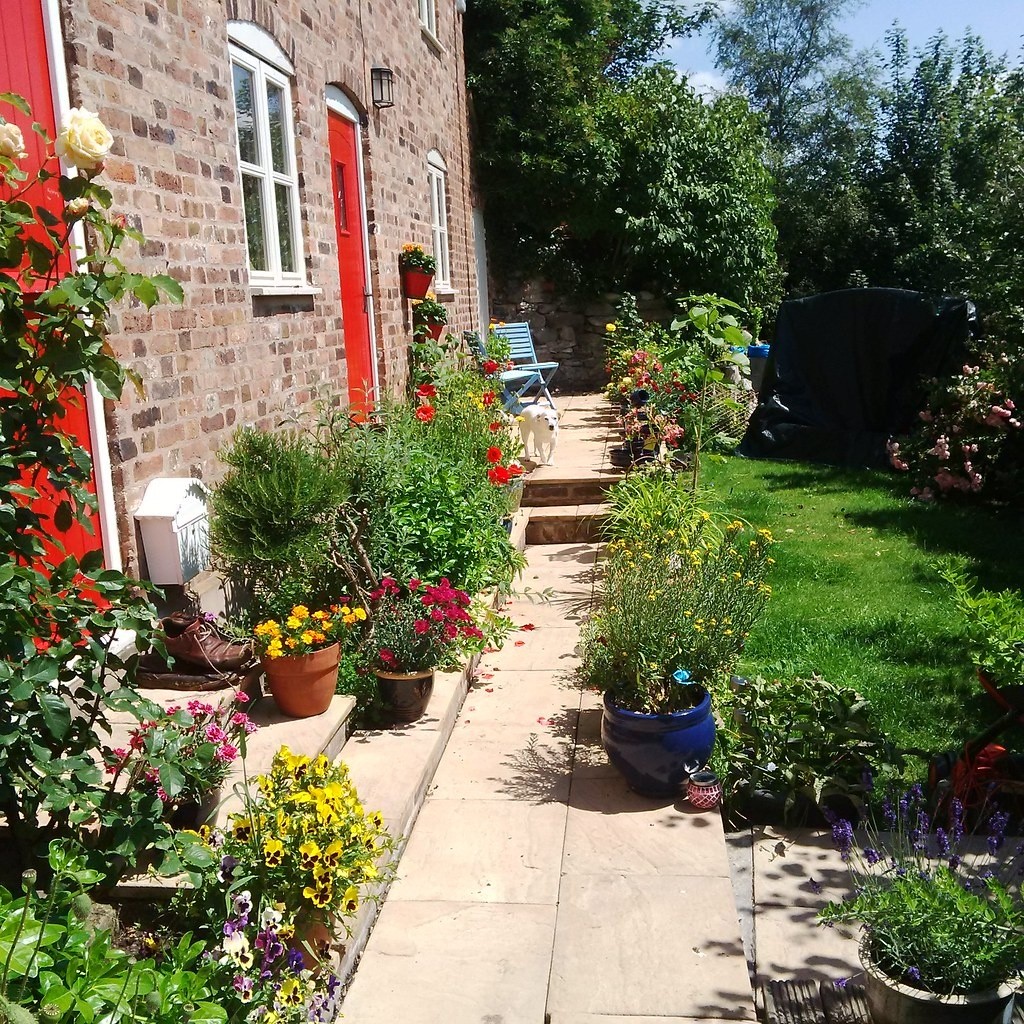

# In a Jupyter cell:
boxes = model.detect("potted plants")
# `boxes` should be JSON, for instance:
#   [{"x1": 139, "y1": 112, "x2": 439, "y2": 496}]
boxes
[{"x1": 728, "y1": 675, "x2": 907, "y2": 826}]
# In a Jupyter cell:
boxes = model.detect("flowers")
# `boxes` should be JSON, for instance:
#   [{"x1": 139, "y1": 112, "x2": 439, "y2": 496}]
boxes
[
  {"x1": 257, "y1": 572, "x2": 485, "y2": 680},
  {"x1": 888, "y1": 343, "x2": 1024, "y2": 502},
  {"x1": 395, "y1": 242, "x2": 437, "y2": 272},
  {"x1": 801, "y1": 785, "x2": 1015, "y2": 992},
  {"x1": 580, "y1": 517, "x2": 787, "y2": 709},
  {"x1": 417, "y1": 357, "x2": 527, "y2": 488},
  {"x1": 602, "y1": 319, "x2": 696, "y2": 440},
  {"x1": 2, "y1": 103, "x2": 167, "y2": 339},
  {"x1": 410, "y1": 290, "x2": 447, "y2": 321},
  {"x1": 100, "y1": 694, "x2": 388, "y2": 1023}
]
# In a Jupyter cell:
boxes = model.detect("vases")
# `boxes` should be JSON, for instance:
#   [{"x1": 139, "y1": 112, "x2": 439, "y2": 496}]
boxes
[
  {"x1": 860, "y1": 913, "x2": 1019, "y2": 1022},
  {"x1": 402, "y1": 258, "x2": 438, "y2": 302},
  {"x1": 232, "y1": 869, "x2": 358, "y2": 976},
  {"x1": 149, "y1": 751, "x2": 239, "y2": 831},
  {"x1": 595, "y1": 682, "x2": 717, "y2": 802},
  {"x1": 363, "y1": 654, "x2": 437, "y2": 723},
  {"x1": 691, "y1": 767, "x2": 728, "y2": 812},
  {"x1": 608, "y1": 437, "x2": 654, "y2": 469},
  {"x1": 269, "y1": 635, "x2": 345, "y2": 715},
  {"x1": 413, "y1": 318, "x2": 452, "y2": 343}
]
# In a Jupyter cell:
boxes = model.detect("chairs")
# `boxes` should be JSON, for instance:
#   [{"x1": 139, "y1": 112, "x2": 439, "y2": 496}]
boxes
[
  {"x1": 466, "y1": 333, "x2": 538, "y2": 414},
  {"x1": 491, "y1": 321, "x2": 559, "y2": 409}
]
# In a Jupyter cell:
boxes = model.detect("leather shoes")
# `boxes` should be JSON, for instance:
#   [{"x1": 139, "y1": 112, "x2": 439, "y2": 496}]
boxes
[{"x1": 152, "y1": 611, "x2": 261, "y2": 673}]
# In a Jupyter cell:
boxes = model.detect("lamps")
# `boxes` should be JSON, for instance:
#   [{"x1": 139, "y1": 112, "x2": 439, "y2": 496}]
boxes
[{"x1": 368, "y1": 63, "x2": 393, "y2": 113}]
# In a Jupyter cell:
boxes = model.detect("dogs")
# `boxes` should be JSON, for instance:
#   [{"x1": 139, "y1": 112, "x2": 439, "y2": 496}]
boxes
[{"x1": 518, "y1": 405, "x2": 562, "y2": 465}]
